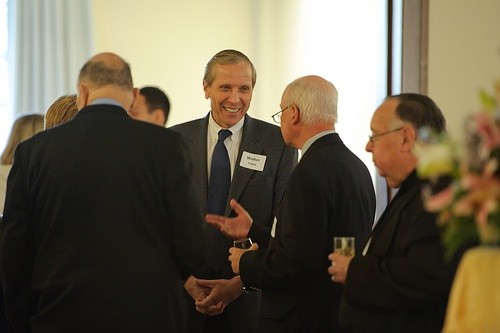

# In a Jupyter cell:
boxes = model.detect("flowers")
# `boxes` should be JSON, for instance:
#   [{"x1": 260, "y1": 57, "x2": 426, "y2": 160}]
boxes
[{"x1": 412, "y1": 81, "x2": 500, "y2": 262}]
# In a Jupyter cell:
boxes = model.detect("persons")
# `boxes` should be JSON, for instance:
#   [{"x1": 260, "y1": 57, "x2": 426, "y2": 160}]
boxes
[
  {"x1": 167, "y1": 50, "x2": 299, "y2": 333},
  {"x1": 329, "y1": 92, "x2": 479, "y2": 333},
  {"x1": 206, "y1": 76, "x2": 376, "y2": 333},
  {"x1": 0, "y1": 53, "x2": 214, "y2": 333}
]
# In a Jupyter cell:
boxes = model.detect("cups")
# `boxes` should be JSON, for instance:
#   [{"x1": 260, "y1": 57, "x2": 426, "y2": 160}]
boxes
[
  {"x1": 334, "y1": 237, "x2": 355, "y2": 257},
  {"x1": 233, "y1": 238, "x2": 252, "y2": 250}
]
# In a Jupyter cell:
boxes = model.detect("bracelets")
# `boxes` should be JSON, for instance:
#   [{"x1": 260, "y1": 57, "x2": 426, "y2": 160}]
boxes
[{"x1": 237, "y1": 275, "x2": 249, "y2": 294}]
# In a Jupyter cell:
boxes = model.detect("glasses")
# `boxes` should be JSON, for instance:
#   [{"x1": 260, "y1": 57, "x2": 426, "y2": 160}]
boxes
[
  {"x1": 272, "y1": 105, "x2": 291, "y2": 123},
  {"x1": 368, "y1": 127, "x2": 403, "y2": 145}
]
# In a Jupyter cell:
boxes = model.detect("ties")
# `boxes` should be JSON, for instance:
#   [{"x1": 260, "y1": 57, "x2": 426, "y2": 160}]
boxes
[{"x1": 208, "y1": 129, "x2": 233, "y2": 217}]
